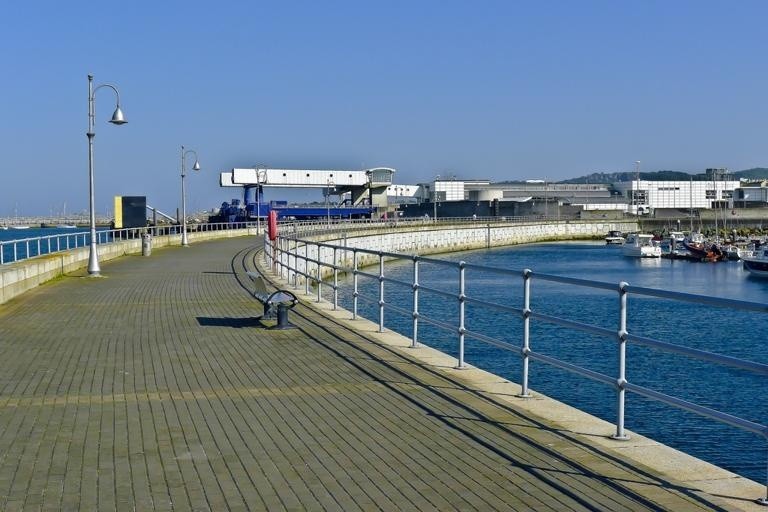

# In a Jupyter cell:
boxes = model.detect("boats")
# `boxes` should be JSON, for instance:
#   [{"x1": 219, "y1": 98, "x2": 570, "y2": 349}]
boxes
[{"x1": 605, "y1": 231, "x2": 768, "y2": 277}]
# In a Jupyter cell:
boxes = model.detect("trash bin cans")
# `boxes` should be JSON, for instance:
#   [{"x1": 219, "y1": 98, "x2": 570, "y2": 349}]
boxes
[{"x1": 142, "y1": 234, "x2": 151, "y2": 256}]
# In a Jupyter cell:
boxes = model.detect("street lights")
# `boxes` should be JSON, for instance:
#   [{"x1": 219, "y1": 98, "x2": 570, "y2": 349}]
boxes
[
  {"x1": 257, "y1": 168, "x2": 269, "y2": 236},
  {"x1": 181, "y1": 145, "x2": 201, "y2": 245},
  {"x1": 327, "y1": 182, "x2": 336, "y2": 229},
  {"x1": 87, "y1": 74, "x2": 129, "y2": 275}
]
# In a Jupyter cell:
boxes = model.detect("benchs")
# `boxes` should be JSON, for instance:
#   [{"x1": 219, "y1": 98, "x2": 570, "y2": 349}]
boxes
[{"x1": 246, "y1": 271, "x2": 301, "y2": 330}]
